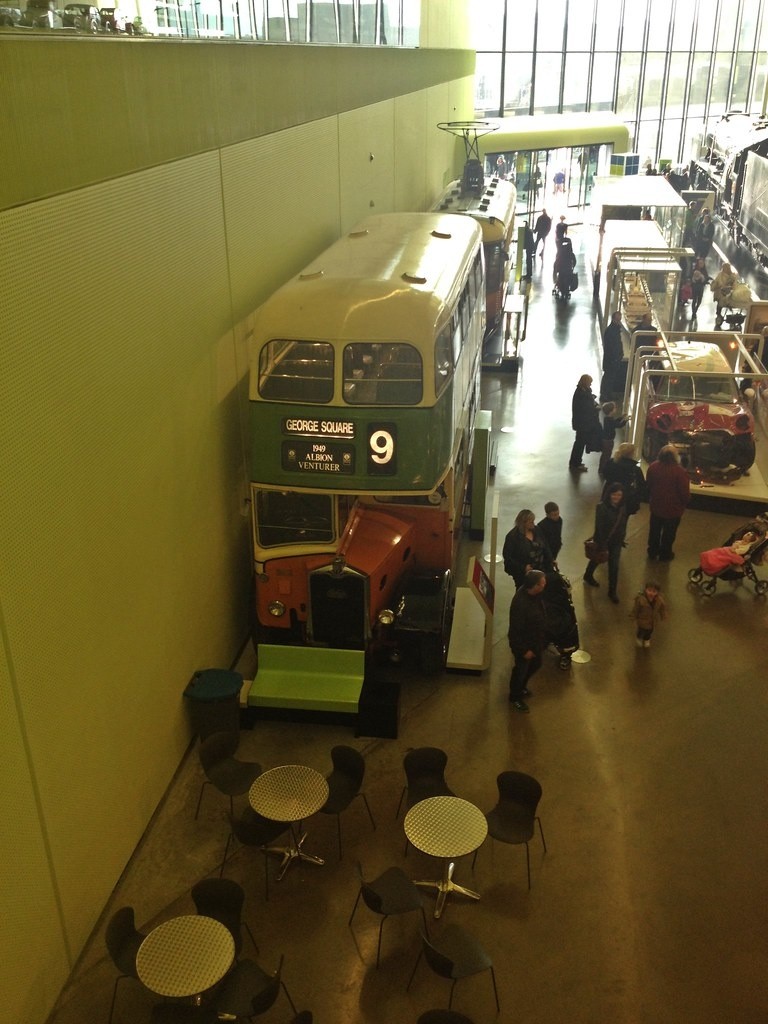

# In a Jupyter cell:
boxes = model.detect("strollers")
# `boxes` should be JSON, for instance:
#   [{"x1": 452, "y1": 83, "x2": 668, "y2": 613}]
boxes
[
  {"x1": 552, "y1": 265, "x2": 579, "y2": 300},
  {"x1": 715, "y1": 286, "x2": 752, "y2": 331},
  {"x1": 688, "y1": 511, "x2": 768, "y2": 596}
]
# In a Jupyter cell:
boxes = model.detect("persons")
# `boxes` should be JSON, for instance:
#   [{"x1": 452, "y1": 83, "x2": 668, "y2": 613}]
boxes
[
  {"x1": 553, "y1": 169, "x2": 565, "y2": 195},
  {"x1": 729, "y1": 531, "x2": 757, "y2": 554},
  {"x1": 577, "y1": 149, "x2": 588, "y2": 179},
  {"x1": 569, "y1": 375, "x2": 601, "y2": 472},
  {"x1": 533, "y1": 167, "x2": 543, "y2": 197},
  {"x1": 679, "y1": 259, "x2": 739, "y2": 329},
  {"x1": 628, "y1": 581, "x2": 666, "y2": 648},
  {"x1": 598, "y1": 402, "x2": 631, "y2": 479},
  {"x1": 494, "y1": 155, "x2": 505, "y2": 179},
  {"x1": 646, "y1": 445, "x2": 690, "y2": 562},
  {"x1": 646, "y1": 159, "x2": 708, "y2": 194},
  {"x1": 599, "y1": 311, "x2": 624, "y2": 401},
  {"x1": 507, "y1": 570, "x2": 546, "y2": 713},
  {"x1": 503, "y1": 502, "x2": 563, "y2": 593},
  {"x1": 519, "y1": 208, "x2": 576, "y2": 301},
  {"x1": 583, "y1": 443, "x2": 647, "y2": 604},
  {"x1": 682, "y1": 201, "x2": 715, "y2": 257},
  {"x1": 632, "y1": 313, "x2": 657, "y2": 355}
]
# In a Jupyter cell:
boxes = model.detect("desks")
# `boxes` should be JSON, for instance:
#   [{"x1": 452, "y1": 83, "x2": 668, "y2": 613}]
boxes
[
  {"x1": 404, "y1": 796, "x2": 488, "y2": 919},
  {"x1": 136, "y1": 915, "x2": 235, "y2": 1006},
  {"x1": 249, "y1": 765, "x2": 330, "y2": 881},
  {"x1": 183, "y1": 668, "x2": 244, "y2": 748}
]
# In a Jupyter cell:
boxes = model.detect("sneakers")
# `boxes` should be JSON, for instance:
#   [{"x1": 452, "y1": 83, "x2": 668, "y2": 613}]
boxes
[
  {"x1": 644, "y1": 639, "x2": 651, "y2": 648},
  {"x1": 635, "y1": 635, "x2": 643, "y2": 647}
]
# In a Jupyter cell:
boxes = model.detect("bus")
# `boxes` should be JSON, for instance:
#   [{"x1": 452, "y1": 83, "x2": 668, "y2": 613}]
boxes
[
  {"x1": 432, "y1": 175, "x2": 518, "y2": 326},
  {"x1": 242, "y1": 210, "x2": 488, "y2": 663}
]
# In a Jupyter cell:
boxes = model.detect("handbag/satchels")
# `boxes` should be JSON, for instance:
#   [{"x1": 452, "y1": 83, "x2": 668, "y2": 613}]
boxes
[{"x1": 584, "y1": 541, "x2": 608, "y2": 564}]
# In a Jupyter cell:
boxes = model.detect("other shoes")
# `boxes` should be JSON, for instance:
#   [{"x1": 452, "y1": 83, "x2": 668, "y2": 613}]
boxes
[
  {"x1": 658, "y1": 552, "x2": 675, "y2": 560},
  {"x1": 521, "y1": 689, "x2": 534, "y2": 697},
  {"x1": 607, "y1": 591, "x2": 619, "y2": 604},
  {"x1": 511, "y1": 699, "x2": 528, "y2": 711},
  {"x1": 583, "y1": 573, "x2": 600, "y2": 587}
]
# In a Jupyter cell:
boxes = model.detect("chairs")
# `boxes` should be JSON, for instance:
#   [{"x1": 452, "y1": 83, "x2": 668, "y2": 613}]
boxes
[
  {"x1": 104, "y1": 906, "x2": 166, "y2": 1024},
  {"x1": 150, "y1": 954, "x2": 313, "y2": 1024},
  {"x1": 349, "y1": 861, "x2": 431, "y2": 969},
  {"x1": 406, "y1": 924, "x2": 501, "y2": 1012},
  {"x1": 295, "y1": 745, "x2": 375, "y2": 862},
  {"x1": 396, "y1": 747, "x2": 457, "y2": 858},
  {"x1": 194, "y1": 728, "x2": 262, "y2": 839},
  {"x1": 417, "y1": 1009, "x2": 474, "y2": 1024},
  {"x1": 471, "y1": 771, "x2": 547, "y2": 891},
  {"x1": 219, "y1": 805, "x2": 302, "y2": 902},
  {"x1": 190, "y1": 878, "x2": 260, "y2": 964}
]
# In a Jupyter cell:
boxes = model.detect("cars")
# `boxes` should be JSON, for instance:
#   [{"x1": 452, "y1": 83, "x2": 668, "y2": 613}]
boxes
[{"x1": 642, "y1": 341, "x2": 757, "y2": 472}]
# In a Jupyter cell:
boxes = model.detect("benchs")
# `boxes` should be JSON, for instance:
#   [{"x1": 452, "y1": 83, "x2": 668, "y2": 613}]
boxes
[
  {"x1": 267, "y1": 342, "x2": 424, "y2": 404},
  {"x1": 248, "y1": 644, "x2": 365, "y2": 725}
]
